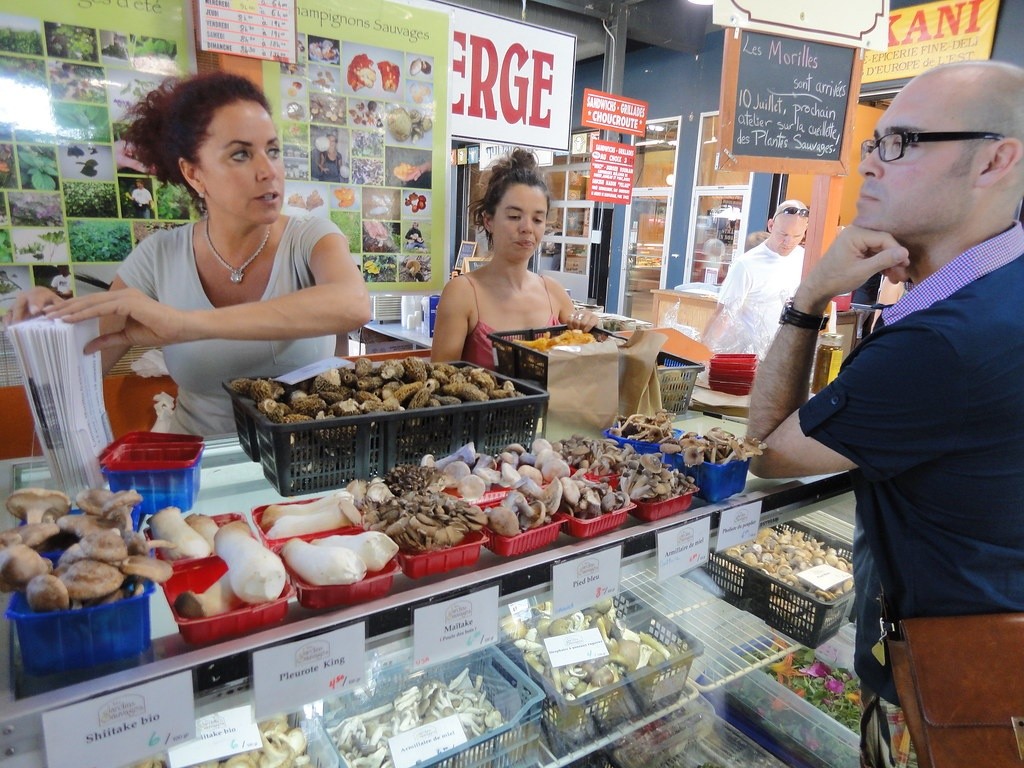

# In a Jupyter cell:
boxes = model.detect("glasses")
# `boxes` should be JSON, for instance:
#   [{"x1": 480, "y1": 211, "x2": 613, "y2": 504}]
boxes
[
  {"x1": 862, "y1": 131, "x2": 1003, "y2": 163},
  {"x1": 774, "y1": 206, "x2": 809, "y2": 220}
]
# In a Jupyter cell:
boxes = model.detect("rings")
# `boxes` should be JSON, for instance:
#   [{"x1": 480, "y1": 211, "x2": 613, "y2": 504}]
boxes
[{"x1": 568, "y1": 313, "x2": 584, "y2": 320}]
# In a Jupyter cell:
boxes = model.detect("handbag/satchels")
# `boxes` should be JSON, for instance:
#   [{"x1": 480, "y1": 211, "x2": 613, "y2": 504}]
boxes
[{"x1": 886, "y1": 611, "x2": 1023, "y2": 768}]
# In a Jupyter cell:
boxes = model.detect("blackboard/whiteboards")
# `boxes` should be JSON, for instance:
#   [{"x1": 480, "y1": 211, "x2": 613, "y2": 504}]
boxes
[
  {"x1": 465, "y1": 257, "x2": 493, "y2": 273},
  {"x1": 712, "y1": 26, "x2": 866, "y2": 176},
  {"x1": 454, "y1": 240, "x2": 477, "y2": 270}
]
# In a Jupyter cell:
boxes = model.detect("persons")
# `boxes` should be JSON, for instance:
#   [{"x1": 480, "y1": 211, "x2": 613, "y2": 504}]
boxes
[
  {"x1": 49, "y1": 265, "x2": 74, "y2": 300},
  {"x1": 745, "y1": 59, "x2": 1023, "y2": 768},
  {"x1": 125, "y1": 179, "x2": 153, "y2": 219},
  {"x1": 431, "y1": 147, "x2": 599, "y2": 369},
  {"x1": 1, "y1": 71, "x2": 370, "y2": 440},
  {"x1": 405, "y1": 221, "x2": 426, "y2": 250},
  {"x1": 317, "y1": 131, "x2": 343, "y2": 183},
  {"x1": 696, "y1": 199, "x2": 810, "y2": 361}
]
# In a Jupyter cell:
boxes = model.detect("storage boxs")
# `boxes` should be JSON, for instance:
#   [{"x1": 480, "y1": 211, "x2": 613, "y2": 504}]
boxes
[
  {"x1": 6, "y1": 324, "x2": 758, "y2": 673},
  {"x1": 289, "y1": 521, "x2": 863, "y2": 768}
]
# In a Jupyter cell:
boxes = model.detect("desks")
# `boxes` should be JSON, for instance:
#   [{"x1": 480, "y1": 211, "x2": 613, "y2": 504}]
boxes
[{"x1": 362, "y1": 323, "x2": 432, "y2": 348}]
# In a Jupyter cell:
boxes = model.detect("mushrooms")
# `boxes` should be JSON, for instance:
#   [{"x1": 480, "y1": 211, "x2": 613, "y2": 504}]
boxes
[
  {"x1": 0, "y1": 357, "x2": 854, "y2": 768},
  {"x1": 387, "y1": 107, "x2": 433, "y2": 144}
]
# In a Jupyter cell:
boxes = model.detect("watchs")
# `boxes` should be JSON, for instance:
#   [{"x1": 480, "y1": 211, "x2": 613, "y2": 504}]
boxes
[{"x1": 777, "y1": 296, "x2": 829, "y2": 332}]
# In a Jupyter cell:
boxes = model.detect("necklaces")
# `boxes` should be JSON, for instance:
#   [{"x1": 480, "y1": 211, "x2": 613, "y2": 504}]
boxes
[{"x1": 204, "y1": 215, "x2": 270, "y2": 282}]
